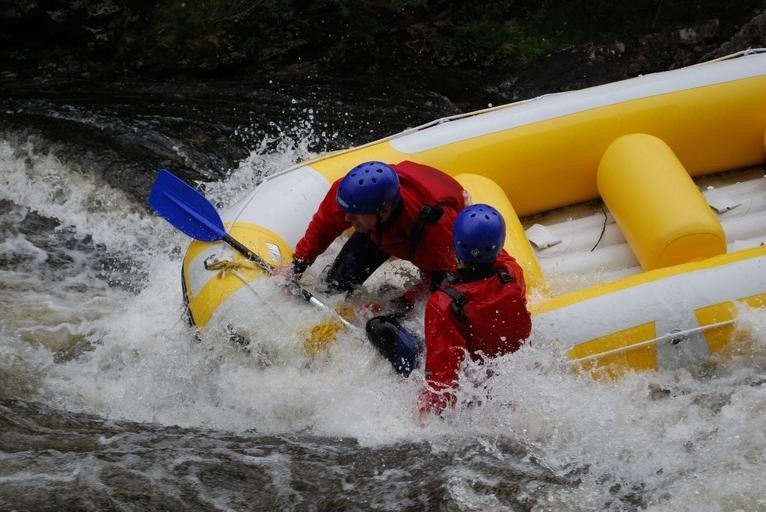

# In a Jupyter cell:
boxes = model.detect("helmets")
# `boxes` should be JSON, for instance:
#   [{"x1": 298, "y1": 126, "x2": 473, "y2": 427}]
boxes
[
  {"x1": 334, "y1": 159, "x2": 403, "y2": 218},
  {"x1": 451, "y1": 202, "x2": 507, "y2": 266}
]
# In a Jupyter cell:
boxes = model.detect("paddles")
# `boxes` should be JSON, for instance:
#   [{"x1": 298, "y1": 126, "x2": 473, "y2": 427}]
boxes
[{"x1": 149, "y1": 170, "x2": 359, "y2": 333}]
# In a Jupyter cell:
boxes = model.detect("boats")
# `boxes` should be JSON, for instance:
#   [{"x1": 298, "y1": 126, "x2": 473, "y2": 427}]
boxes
[{"x1": 174, "y1": 47, "x2": 766, "y2": 404}]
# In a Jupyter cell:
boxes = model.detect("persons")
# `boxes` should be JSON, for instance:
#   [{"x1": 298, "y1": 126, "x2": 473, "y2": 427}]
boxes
[
  {"x1": 265, "y1": 159, "x2": 472, "y2": 327},
  {"x1": 367, "y1": 188, "x2": 532, "y2": 429}
]
